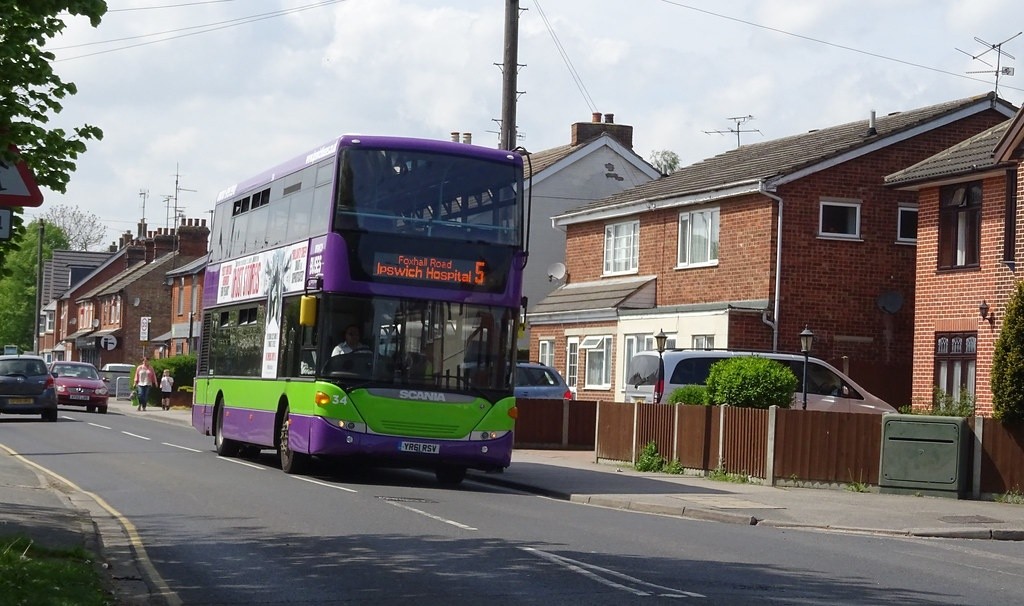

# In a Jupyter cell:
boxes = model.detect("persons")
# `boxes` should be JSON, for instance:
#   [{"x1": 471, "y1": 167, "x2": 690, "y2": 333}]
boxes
[
  {"x1": 331, "y1": 326, "x2": 372, "y2": 357},
  {"x1": 134, "y1": 357, "x2": 158, "y2": 411},
  {"x1": 160, "y1": 369, "x2": 174, "y2": 410}
]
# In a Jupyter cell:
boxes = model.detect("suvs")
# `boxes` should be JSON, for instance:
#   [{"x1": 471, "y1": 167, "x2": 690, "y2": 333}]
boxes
[{"x1": 624, "y1": 347, "x2": 900, "y2": 417}]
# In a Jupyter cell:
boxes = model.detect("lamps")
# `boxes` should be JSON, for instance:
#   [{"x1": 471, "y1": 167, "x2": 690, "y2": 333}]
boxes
[{"x1": 980, "y1": 299, "x2": 993, "y2": 324}]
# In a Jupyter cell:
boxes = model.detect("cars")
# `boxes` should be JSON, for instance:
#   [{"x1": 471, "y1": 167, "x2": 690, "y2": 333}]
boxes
[
  {"x1": 102, "y1": 363, "x2": 136, "y2": 372},
  {"x1": 34, "y1": 362, "x2": 71, "y2": 374},
  {"x1": 0, "y1": 354, "x2": 58, "y2": 422},
  {"x1": 48, "y1": 361, "x2": 111, "y2": 414},
  {"x1": 507, "y1": 359, "x2": 574, "y2": 401}
]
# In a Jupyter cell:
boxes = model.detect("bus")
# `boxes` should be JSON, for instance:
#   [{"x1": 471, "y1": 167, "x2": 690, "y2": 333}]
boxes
[{"x1": 191, "y1": 134, "x2": 532, "y2": 485}]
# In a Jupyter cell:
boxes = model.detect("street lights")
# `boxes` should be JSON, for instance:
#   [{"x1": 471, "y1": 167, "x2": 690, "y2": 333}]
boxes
[
  {"x1": 653, "y1": 328, "x2": 668, "y2": 403},
  {"x1": 799, "y1": 324, "x2": 817, "y2": 410}
]
